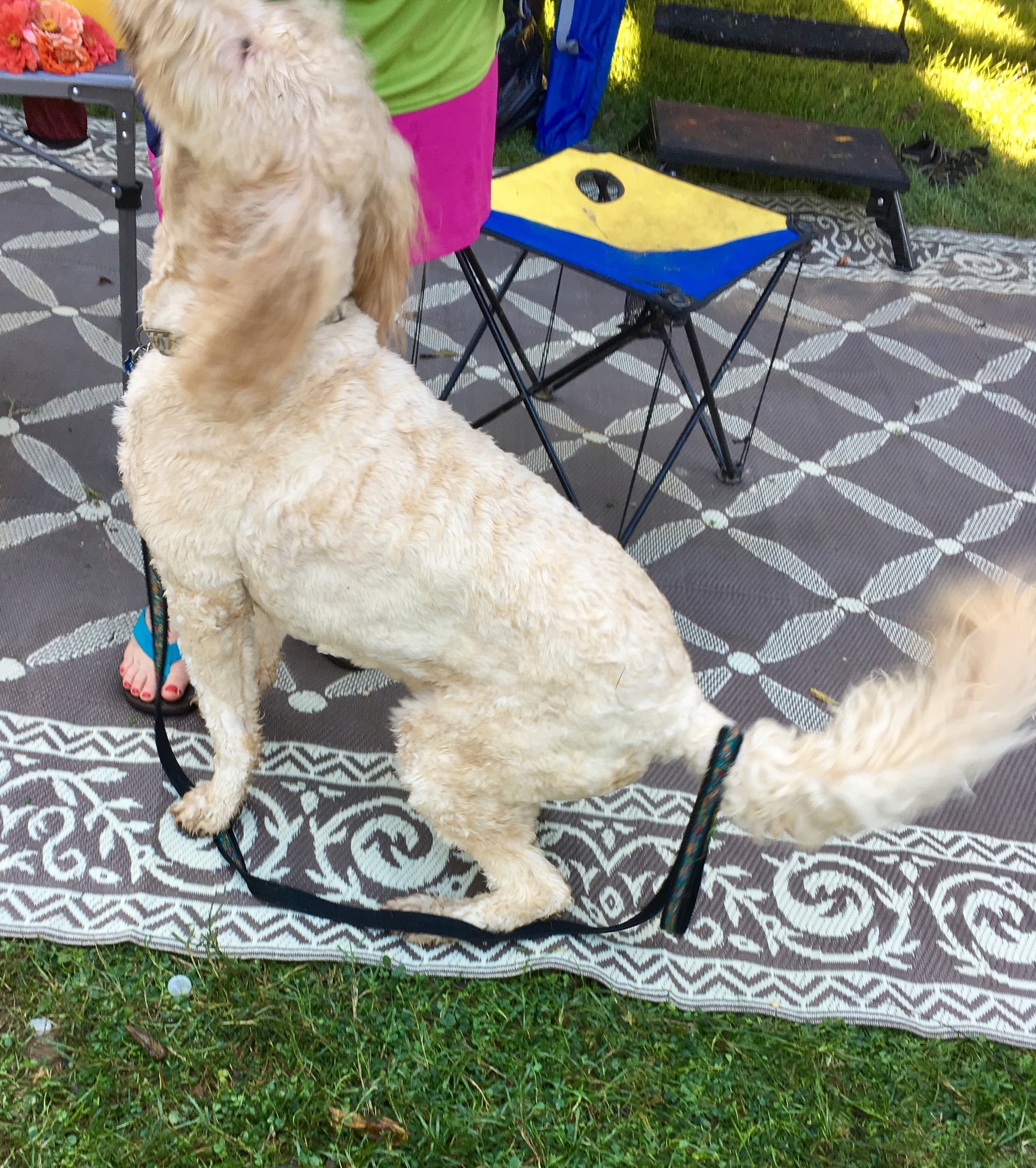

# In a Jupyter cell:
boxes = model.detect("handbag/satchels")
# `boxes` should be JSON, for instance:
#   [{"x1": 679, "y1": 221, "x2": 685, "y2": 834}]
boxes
[{"x1": 495, "y1": 0, "x2": 548, "y2": 133}]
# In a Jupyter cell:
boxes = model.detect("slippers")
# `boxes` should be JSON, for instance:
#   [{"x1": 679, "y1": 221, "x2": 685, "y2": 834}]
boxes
[
  {"x1": 313, "y1": 643, "x2": 366, "y2": 671},
  {"x1": 122, "y1": 605, "x2": 197, "y2": 716}
]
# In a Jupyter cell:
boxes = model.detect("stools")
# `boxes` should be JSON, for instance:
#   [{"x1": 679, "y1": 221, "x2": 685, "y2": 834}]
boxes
[{"x1": 437, "y1": 148, "x2": 812, "y2": 552}]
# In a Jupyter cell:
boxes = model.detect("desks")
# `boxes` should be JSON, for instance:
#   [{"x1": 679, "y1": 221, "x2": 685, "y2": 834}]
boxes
[{"x1": 0, "y1": 50, "x2": 139, "y2": 396}]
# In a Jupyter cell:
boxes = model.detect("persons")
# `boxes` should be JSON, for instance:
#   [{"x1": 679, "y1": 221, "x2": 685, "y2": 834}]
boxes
[{"x1": 117, "y1": 1, "x2": 503, "y2": 726}]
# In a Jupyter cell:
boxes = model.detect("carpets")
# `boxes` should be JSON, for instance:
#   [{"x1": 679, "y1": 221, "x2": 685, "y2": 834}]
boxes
[{"x1": 0, "y1": 107, "x2": 1036, "y2": 1052}]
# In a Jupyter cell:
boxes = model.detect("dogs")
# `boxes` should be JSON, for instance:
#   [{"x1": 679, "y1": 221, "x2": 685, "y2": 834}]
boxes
[{"x1": 114, "y1": 3, "x2": 1035, "y2": 936}]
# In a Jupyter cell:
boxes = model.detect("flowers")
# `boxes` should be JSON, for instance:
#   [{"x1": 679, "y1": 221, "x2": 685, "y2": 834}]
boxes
[{"x1": 0, "y1": 0, "x2": 118, "y2": 77}]
